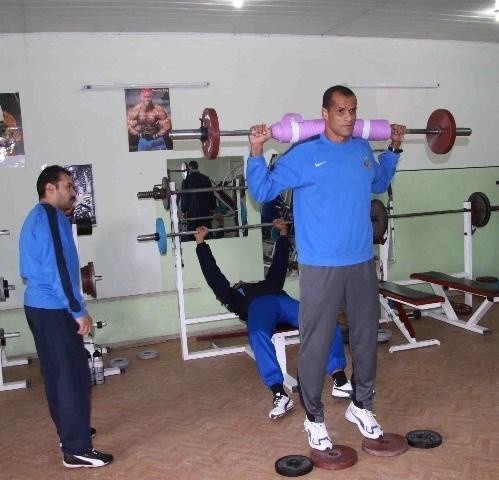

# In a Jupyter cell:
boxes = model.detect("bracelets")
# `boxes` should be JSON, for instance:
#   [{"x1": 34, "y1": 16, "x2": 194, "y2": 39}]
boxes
[{"x1": 387, "y1": 142, "x2": 406, "y2": 156}]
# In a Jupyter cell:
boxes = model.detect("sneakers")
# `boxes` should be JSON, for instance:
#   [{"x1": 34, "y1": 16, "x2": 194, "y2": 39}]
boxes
[
  {"x1": 331, "y1": 379, "x2": 375, "y2": 399},
  {"x1": 303, "y1": 414, "x2": 333, "y2": 451},
  {"x1": 59, "y1": 428, "x2": 96, "y2": 451},
  {"x1": 345, "y1": 401, "x2": 383, "y2": 440},
  {"x1": 63, "y1": 449, "x2": 113, "y2": 468},
  {"x1": 269, "y1": 393, "x2": 295, "y2": 420}
]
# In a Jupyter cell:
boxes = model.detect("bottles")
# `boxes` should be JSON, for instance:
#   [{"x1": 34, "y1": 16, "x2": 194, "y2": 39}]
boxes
[
  {"x1": 87, "y1": 353, "x2": 96, "y2": 385},
  {"x1": 93, "y1": 351, "x2": 105, "y2": 385}
]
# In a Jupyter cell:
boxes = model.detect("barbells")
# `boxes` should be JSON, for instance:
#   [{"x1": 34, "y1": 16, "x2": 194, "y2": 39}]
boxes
[
  {"x1": 370, "y1": 192, "x2": 499, "y2": 245},
  {"x1": 169, "y1": 107, "x2": 471, "y2": 160},
  {"x1": 137, "y1": 177, "x2": 248, "y2": 210},
  {"x1": 137, "y1": 218, "x2": 294, "y2": 254}
]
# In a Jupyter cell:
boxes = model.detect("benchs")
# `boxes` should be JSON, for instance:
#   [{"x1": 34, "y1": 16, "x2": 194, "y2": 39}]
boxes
[
  {"x1": 376, "y1": 277, "x2": 447, "y2": 355},
  {"x1": 236, "y1": 315, "x2": 301, "y2": 393},
  {"x1": 410, "y1": 268, "x2": 499, "y2": 335}
]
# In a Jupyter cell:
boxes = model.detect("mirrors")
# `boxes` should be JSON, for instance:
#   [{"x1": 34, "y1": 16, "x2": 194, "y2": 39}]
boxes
[
  {"x1": 261, "y1": 152, "x2": 301, "y2": 279},
  {"x1": 167, "y1": 156, "x2": 248, "y2": 244}
]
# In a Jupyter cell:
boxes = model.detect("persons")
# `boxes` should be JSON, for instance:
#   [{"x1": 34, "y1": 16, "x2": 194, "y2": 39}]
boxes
[
  {"x1": 245, "y1": 85, "x2": 407, "y2": 451},
  {"x1": 178, "y1": 161, "x2": 215, "y2": 239},
  {"x1": 190, "y1": 217, "x2": 377, "y2": 421},
  {"x1": 17, "y1": 166, "x2": 112, "y2": 472},
  {"x1": 127, "y1": 88, "x2": 171, "y2": 151}
]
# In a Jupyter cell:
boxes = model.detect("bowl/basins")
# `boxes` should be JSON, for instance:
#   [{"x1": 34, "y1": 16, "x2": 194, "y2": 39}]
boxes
[{"x1": 377, "y1": 329, "x2": 393, "y2": 342}]
